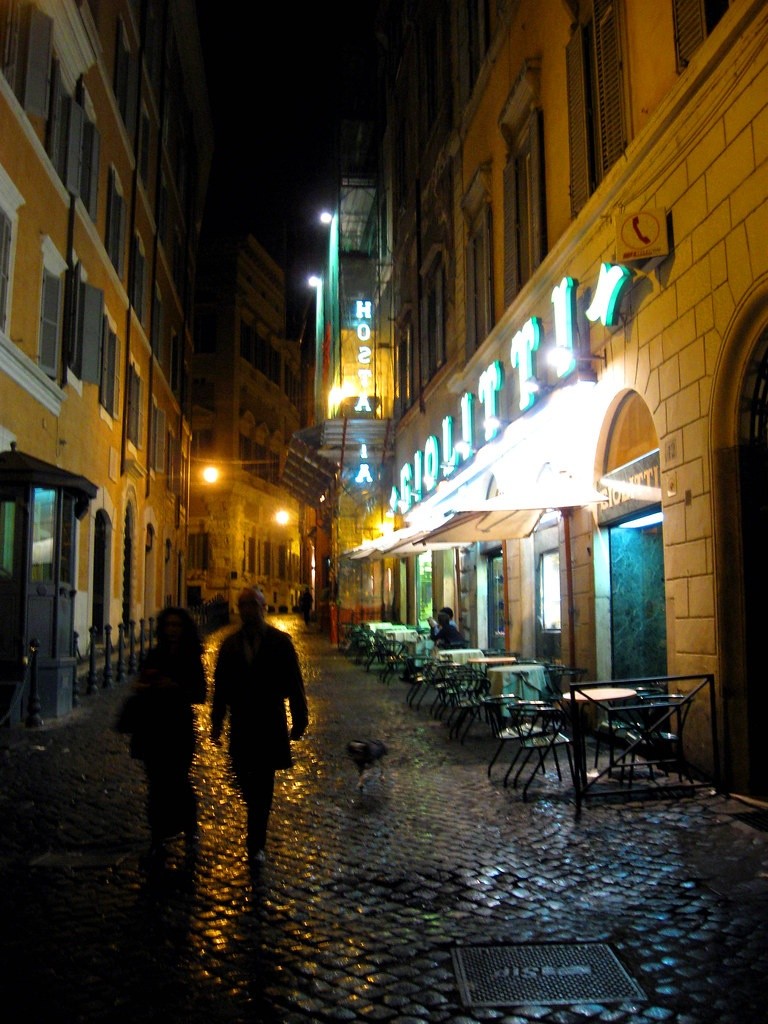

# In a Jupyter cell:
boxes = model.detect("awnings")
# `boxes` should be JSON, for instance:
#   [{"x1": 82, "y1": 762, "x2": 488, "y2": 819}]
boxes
[{"x1": 345, "y1": 473, "x2": 610, "y2": 561}]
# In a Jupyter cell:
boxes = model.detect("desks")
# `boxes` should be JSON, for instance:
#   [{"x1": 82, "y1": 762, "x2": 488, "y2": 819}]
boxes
[
  {"x1": 487, "y1": 666, "x2": 545, "y2": 733},
  {"x1": 563, "y1": 686, "x2": 635, "y2": 780},
  {"x1": 467, "y1": 657, "x2": 517, "y2": 722},
  {"x1": 435, "y1": 648, "x2": 484, "y2": 701},
  {"x1": 404, "y1": 638, "x2": 435, "y2": 667},
  {"x1": 376, "y1": 625, "x2": 408, "y2": 657},
  {"x1": 385, "y1": 630, "x2": 419, "y2": 655},
  {"x1": 365, "y1": 620, "x2": 392, "y2": 657}
]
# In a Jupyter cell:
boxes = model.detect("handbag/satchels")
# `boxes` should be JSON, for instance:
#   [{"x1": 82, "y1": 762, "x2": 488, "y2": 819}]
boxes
[{"x1": 112, "y1": 694, "x2": 148, "y2": 759}]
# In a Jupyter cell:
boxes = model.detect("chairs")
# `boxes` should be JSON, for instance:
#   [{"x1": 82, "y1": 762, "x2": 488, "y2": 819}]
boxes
[{"x1": 346, "y1": 616, "x2": 717, "y2": 805}]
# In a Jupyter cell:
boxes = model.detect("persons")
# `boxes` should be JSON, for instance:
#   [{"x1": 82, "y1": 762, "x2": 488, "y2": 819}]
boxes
[
  {"x1": 301, "y1": 587, "x2": 313, "y2": 625},
  {"x1": 209, "y1": 587, "x2": 308, "y2": 871},
  {"x1": 113, "y1": 605, "x2": 206, "y2": 852},
  {"x1": 429, "y1": 607, "x2": 465, "y2": 650}
]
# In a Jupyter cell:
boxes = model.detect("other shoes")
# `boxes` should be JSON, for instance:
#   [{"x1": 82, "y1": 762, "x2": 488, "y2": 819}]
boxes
[{"x1": 245, "y1": 845, "x2": 268, "y2": 867}]
